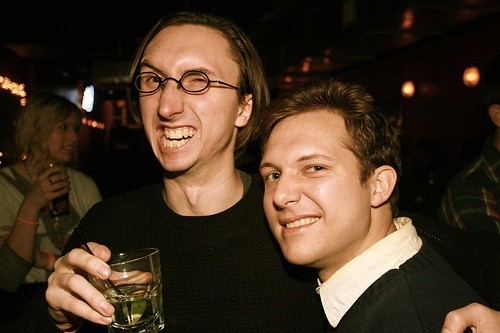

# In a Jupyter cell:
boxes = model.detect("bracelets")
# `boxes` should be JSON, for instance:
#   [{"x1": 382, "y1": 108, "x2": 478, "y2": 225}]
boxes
[
  {"x1": 14, "y1": 216, "x2": 40, "y2": 229},
  {"x1": 64, "y1": 320, "x2": 84, "y2": 332}
]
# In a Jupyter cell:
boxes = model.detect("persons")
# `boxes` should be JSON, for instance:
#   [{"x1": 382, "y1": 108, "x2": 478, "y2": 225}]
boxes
[{"x1": 0, "y1": 11, "x2": 499, "y2": 332}]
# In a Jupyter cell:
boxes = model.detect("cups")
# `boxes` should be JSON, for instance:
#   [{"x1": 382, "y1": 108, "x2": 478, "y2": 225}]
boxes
[
  {"x1": 35, "y1": 157, "x2": 71, "y2": 220},
  {"x1": 86, "y1": 247, "x2": 165, "y2": 333}
]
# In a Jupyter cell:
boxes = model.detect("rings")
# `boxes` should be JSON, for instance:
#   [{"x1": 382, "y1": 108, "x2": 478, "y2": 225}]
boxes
[{"x1": 47, "y1": 178, "x2": 53, "y2": 185}]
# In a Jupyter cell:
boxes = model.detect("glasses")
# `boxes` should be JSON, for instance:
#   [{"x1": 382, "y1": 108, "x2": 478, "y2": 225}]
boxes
[{"x1": 134, "y1": 68, "x2": 242, "y2": 92}]
